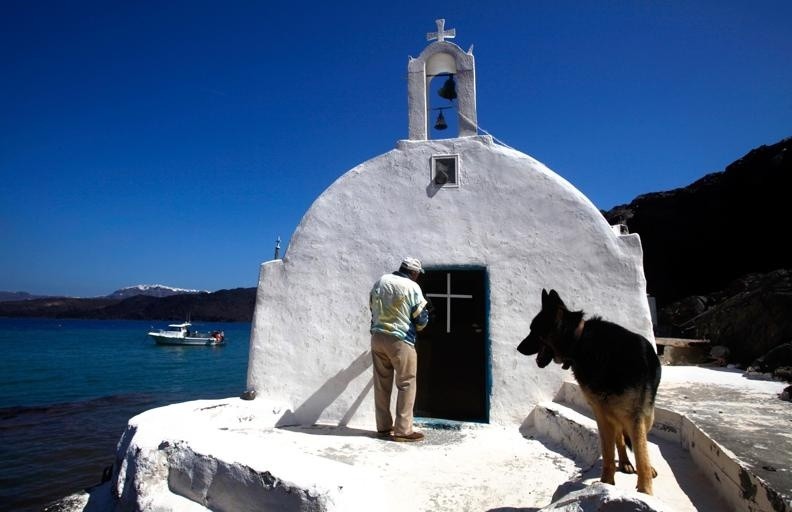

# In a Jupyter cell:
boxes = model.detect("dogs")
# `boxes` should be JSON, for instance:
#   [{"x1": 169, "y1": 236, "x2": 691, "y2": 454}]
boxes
[{"x1": 516, "y1": 289, "x2": 662, "y2": 494}]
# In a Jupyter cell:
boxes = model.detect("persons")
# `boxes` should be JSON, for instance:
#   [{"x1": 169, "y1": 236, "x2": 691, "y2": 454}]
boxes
[{"x1": 369, "y1": 257, "x2": 428, "y2": 442}]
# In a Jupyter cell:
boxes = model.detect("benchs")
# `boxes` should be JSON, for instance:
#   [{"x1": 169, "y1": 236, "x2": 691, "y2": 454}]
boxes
[{"x1": 655, "y1": 337, "x2": 711, "y2": 365}]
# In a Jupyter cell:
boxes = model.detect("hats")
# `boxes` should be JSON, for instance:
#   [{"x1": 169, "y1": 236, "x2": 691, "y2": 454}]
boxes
[{"x1": 401, "y1": 257, "x2": 425, "y2": 274}]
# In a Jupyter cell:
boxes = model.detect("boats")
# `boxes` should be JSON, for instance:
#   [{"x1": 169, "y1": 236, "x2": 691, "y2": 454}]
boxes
[{"x1": 148, "y1": 322, "x2": 224, "y2": 345}]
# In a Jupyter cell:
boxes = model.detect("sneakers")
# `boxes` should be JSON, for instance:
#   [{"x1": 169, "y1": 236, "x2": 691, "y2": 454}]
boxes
[
  {"x1": 378, "y1": 427, "x2": 394, "y2": 436},
  {"x1": 394, "y1": 432, "x2": 424, "y2": 442}
]
